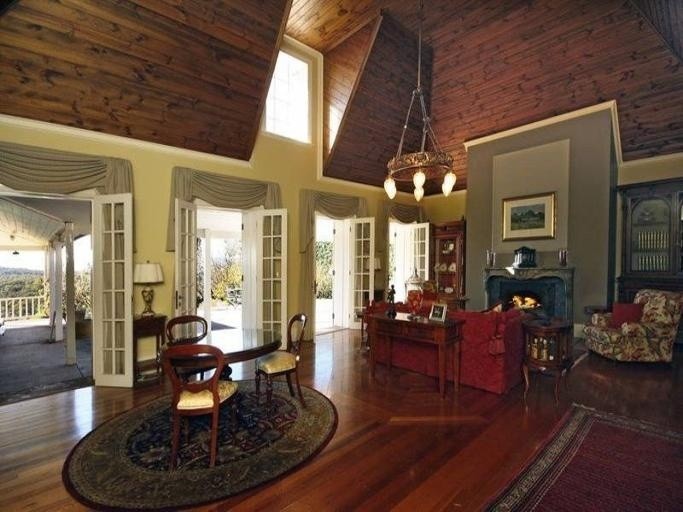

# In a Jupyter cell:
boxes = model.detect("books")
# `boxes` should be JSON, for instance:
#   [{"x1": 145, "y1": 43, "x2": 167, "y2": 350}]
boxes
[{"x1": 638, "y1": 230, "x2": 670, "y2": 272}]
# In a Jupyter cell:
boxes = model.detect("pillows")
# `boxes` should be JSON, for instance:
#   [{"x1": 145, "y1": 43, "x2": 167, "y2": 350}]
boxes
[
  {"x1": 612, "y1": 302, "x2": 643, "y2": 329},
  {"x1": 479, "y1": 301, "x2": 504, "y2": 313}
]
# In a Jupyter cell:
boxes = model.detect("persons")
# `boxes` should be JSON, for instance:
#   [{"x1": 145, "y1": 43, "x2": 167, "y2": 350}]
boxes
[{"x1": 387, "y1": 285, "x2": 395, "y2": 313}]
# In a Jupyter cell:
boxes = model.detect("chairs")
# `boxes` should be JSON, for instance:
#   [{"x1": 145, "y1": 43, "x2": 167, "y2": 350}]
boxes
[
  {"x1": 160, "y1": 344, "x2": 240, "y2": 470},
  {"x1": 582, "y1": 288, "x2": 683, "y2": 364},
  {"x1": 166, "y1": 315, "x2": 233, "y2": 382},
  {"x1": 256, "y1": 312, "x2": 309, "y2": 408}
]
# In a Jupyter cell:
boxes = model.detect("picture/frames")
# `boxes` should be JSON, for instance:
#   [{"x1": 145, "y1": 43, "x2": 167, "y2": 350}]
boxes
[
  {"x1": 501, "y1": 191, "x2": 558, "y2": 243},
  {"x1": 429, "y1": 303, "x2": 448, "y2": 322}
]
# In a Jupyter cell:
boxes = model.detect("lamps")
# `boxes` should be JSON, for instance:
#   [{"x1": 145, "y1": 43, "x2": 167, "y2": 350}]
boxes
[
  {"x1": 366, "y1": 257, "x2": 382, "y2": 290},
  {"x1": 383, "y1": 0, "x2": 458, "y2": 202},
  {"x1": 133, "y1": 260, "x2": 165, "y2": 318}
]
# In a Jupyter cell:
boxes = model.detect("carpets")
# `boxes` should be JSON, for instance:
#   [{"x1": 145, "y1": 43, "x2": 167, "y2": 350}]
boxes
[
  {"x1": 61, "y1": 378, "x2": 339, "y2": 512},
  {"x1": 481, "y1": 401, "x2": 683, "y2": 512}
]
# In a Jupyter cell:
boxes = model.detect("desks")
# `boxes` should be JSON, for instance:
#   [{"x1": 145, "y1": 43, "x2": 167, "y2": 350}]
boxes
[
  {"x1": 520, "y1": 318, "x2": 573, "y2": 408},
  {"x1": 367, "y1": 309, "x2": 466, "y2": 401},
  {"x1": 160, "y1": 328, "x2": 283, "y2": 403},
  {"x1": 133, "y1": 313, "x2": 169, "y2": 383}
]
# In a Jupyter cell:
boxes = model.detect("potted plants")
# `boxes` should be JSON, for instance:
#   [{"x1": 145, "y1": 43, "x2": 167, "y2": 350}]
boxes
[{"x1": 39, "y1": 263, "x2": 93, "y2": 322}]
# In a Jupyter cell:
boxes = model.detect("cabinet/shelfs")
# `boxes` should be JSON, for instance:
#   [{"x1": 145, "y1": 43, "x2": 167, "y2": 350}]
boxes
[{"x1": 430, "y1": 230, "x2": 464, "y2": 297}]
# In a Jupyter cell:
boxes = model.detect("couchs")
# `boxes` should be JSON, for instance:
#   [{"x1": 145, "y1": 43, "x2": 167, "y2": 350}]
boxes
[{"x1": 363, "y1": 300, "x2": 526, "y2": 396}]
